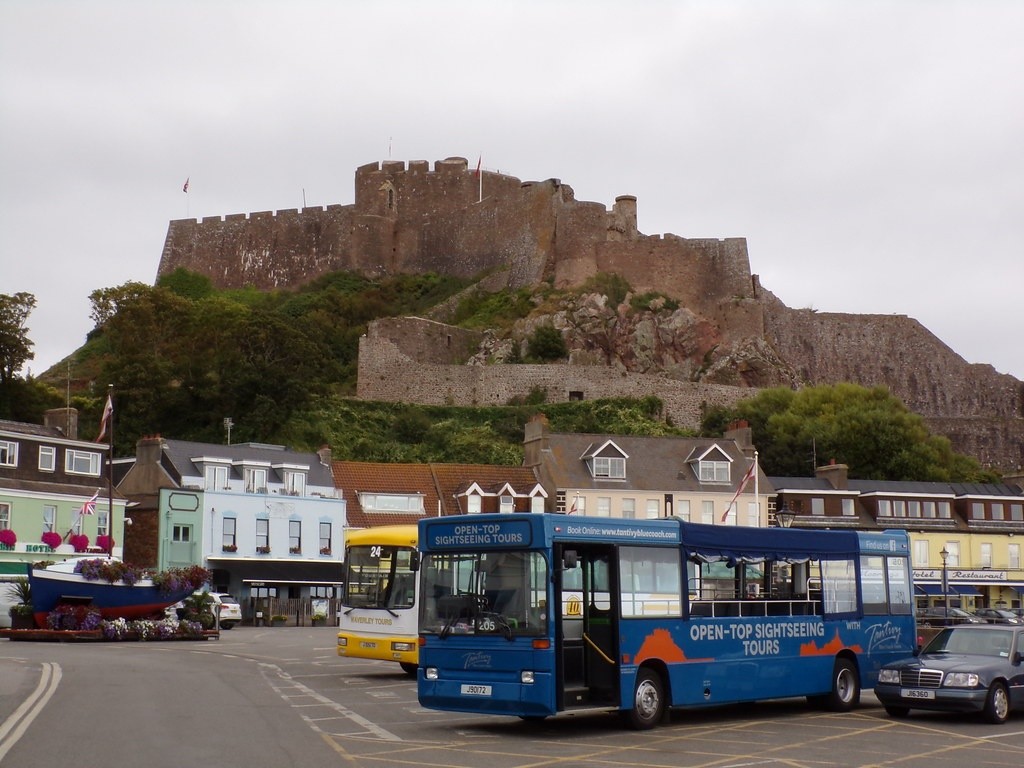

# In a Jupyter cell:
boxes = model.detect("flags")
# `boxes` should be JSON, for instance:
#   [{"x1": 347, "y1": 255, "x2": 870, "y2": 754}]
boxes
[
  {"x1": 568, "y1": 497, "x2": 577, "y2": 514},
  {"x1": 722, "y1": 459, "x2": 755, "y2": 521},
  {"x1": 80, "y1": 490, "x2": 99, "y2": 514},
  {"x1": 97, "y1": 396, "x2": 113, "y2": 443}
]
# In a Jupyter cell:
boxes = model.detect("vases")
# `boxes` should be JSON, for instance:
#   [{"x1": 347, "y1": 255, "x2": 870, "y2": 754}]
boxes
[
  {"x1": 223, "y1": 548, "x2": 237, "y2": 552},
  {"x1": 312, "y1": 620, "x2": 327, "y2": 627},
  {"x1": 271, "y1": 620, "x2": 286, "y2": 627}
]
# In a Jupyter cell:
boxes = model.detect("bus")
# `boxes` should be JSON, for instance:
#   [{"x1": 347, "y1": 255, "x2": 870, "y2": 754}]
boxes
[
  {"x1": 337, "y1": 524, "x2": 700, "y2": 676},
  {"x1": 418, "y1": 513, "x2": 920, "y2": 731}
]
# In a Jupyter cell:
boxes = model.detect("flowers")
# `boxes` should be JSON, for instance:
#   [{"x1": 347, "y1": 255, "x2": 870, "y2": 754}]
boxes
[
  {"x1": 310, "y1": 613, "x2": 327, "y2": 621},
  {"x1": 256, "y1": 545, "x2": 272, "y2": 554},
  {"x1": 223, "y1": 545, "x2": 238, "y2": 552},
  {"x1": 45, "y1": 557, "x2": 214, "y2": 641},
  {"x1": 271, "y1": 614, "x2": 288, "y2": 621},
  {"x1": 289, "y1": 546, "x2": 301, "y2": 554},
  {"x1": 320, "y1": 547, "x2": 332, "y2": 554}
]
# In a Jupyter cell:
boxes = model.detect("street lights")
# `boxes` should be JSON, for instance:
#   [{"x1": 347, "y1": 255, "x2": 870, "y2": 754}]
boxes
[
  {"x1": 775, "y1": 501, "x2": 797, "y2": 591},
  {"x1": 940, "y1": 546, "x2": 949, "y2": 618}
]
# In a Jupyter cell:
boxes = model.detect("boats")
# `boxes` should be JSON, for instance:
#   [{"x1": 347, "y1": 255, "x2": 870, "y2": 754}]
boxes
[{"x1": 27, "y1": 386, "x2": 207, "y2": 630}]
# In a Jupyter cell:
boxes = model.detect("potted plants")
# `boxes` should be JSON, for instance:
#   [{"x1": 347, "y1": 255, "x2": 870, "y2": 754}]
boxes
[
  {"x1": 189, "y1": 592, "x2": 216, "y2": 640},
  {"x1": 4, "y1": 576, "x2": 37, "y2": 641}
]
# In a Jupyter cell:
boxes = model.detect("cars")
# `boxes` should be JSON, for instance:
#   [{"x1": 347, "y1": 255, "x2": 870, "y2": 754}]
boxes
[
  {"x1": 874, "y1": 623, "x2": 1024, "y2": 725},
  {"x1": 164, "y1": 592, "x2": 242, "y2": 630},
  {"x1": 969, "y1": 609, "x2": 1024, "y2": 626},
  {"x1": 916, "y1": 608, "x2": 988, "y2": 629}
]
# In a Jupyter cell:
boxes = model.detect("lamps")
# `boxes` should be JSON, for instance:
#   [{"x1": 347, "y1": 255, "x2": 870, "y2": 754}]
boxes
[
  {"x1": 1008, "y1": 533, "x2": 1014, "y2": 537},
  {"x1": 919, "y1": 530, "x2": 925, "y2": 534},
  {"x1": 825, "y1": 527, "x2": 830, "y2": 530}
]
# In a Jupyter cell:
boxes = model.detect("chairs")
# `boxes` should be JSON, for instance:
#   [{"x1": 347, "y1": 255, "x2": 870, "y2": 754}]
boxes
[
  {"x1": 691, "y1": 589, "x2": 888, "y2": 617},
  {"x1": 989, "y1": 637, "x2": 1009, "y2": 656}
]
